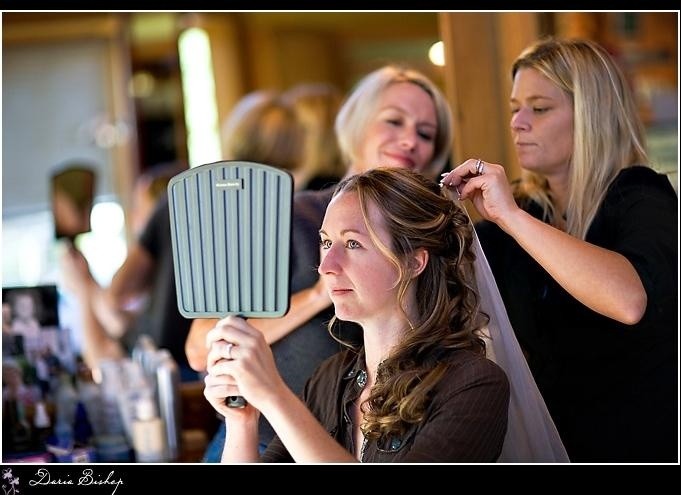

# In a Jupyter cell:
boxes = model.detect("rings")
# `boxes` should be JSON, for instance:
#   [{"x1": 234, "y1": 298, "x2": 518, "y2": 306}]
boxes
[
  {"x1": 227, "y1": 344, "x2": 233, "y2": 358},
  {"x1": 475, "y1": 159, "x2": 483, "y2": 174}
]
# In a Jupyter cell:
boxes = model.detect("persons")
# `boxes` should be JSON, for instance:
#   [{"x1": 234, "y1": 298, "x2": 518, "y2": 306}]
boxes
[
  {"x1": 185, "y1": 65, "x2": 454, "y2": 463},
  {"x1": 2, "y1": 295, "x2": 41, "y2": 407},
  {"x1": 204, "y1": 168, "x2": 508, "y2": 464},
  {"x1": 56, "y1": 89, "x2": 342, "y2": 382},
  {"x1": 439, "y1": 38, "x2": 678, "y2": 462}
]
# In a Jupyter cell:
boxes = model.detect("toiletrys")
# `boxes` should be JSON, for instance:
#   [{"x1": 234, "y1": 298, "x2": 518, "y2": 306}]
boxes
[{"x1": 46, "y1": 337, "x2": 178, "y2": 463}]
